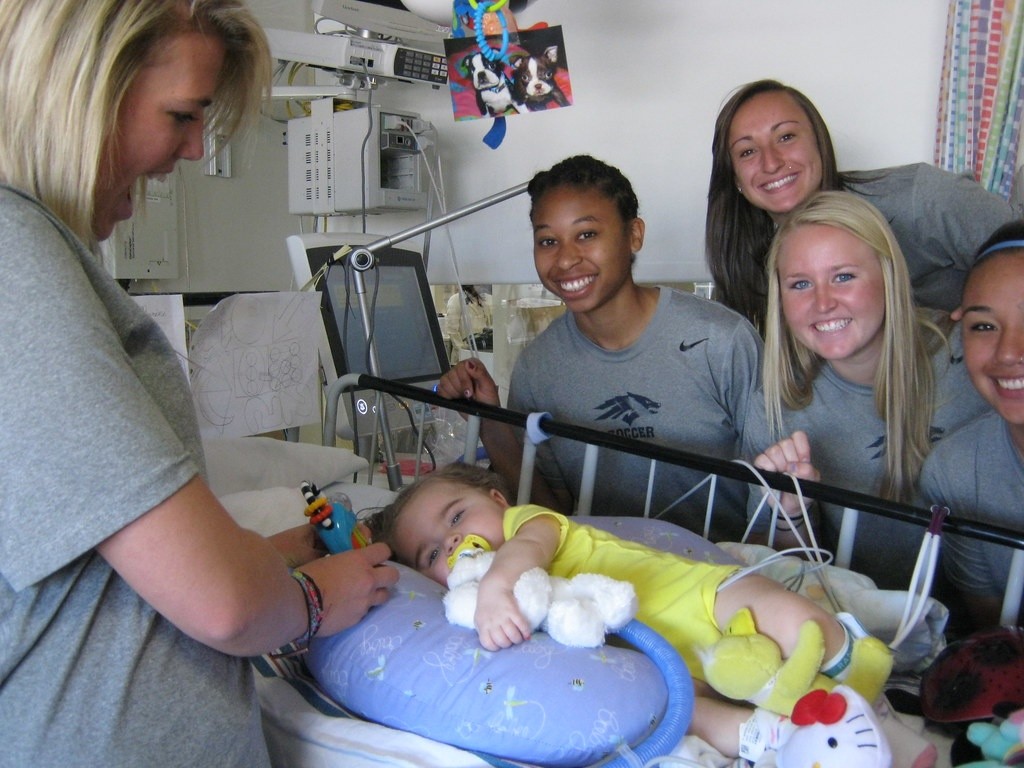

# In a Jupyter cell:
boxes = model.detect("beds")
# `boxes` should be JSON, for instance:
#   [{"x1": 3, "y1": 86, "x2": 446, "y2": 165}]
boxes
[{"x1": 211, "y1": 376, "x2": 1024, "y2": 768}]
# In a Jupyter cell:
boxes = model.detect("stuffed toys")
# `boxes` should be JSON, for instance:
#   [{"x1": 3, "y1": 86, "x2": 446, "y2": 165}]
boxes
[
  {"x1": 956, "y1": 709, "x2": 1024, "y2": 768},
  {"x1": 775, "y1": 686, "x2": 893, "y2": 768},
  {"x1": 442, "y1": 550, "x2": 635, "y2": 648}
]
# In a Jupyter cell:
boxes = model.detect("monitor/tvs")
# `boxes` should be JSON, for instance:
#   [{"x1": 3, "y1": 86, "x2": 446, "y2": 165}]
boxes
[
  {"x1": 284, "y1": 233, "x2": 452, "y2": 439},
  {"x1": 309, "y1": 0, "x2": 452, "y2": 44}
]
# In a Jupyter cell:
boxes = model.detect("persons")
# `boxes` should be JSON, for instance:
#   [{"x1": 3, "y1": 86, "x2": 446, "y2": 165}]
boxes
[
  {"x1": 746, "y1": 190, "x2": 995, "y2": 589},
  {"x1": 0, "y1": 0, "x2": 400, "y2": 767},
  {"x1": 703, "y1": 79, "x2": 1024, "y2": 342},
  {"x1": 438, "y1": 156, "x2": 774, "y2": 543},
  {"x1": 381, "y1": 460, "x2": 939, "y2": 766},
  {"x1": 918, "y1": 221, "x2": 1023, "y2": 625}
]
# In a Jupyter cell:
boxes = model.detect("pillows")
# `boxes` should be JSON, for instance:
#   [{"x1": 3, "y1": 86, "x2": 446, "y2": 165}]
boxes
[{"x1": 204, "y1": 434, "x2": 371, "y2": 500}]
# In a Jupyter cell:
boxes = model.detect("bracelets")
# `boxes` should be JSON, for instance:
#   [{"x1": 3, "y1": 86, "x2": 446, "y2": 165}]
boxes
[
  {"x1": 771, "y1": 504, "x2": 812, "y2": 532},
  {"x1": 293, "y1": 571, "x2": 325, "y2": 639}
]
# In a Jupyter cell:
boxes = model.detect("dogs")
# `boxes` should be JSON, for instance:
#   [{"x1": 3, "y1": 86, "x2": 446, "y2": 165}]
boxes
[{"x1": 464, "y1": 45, "x2": 569, "y2": 118}]
